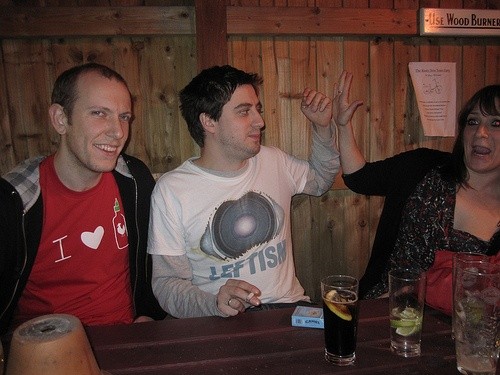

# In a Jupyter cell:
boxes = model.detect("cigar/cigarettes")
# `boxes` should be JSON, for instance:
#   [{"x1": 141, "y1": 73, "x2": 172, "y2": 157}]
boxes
[{"x1": 247, "y1": 290, "x2": 254, "y2": 302}]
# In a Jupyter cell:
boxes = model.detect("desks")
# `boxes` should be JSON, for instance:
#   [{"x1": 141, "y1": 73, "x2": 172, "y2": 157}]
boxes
[{"x1": 82, "y1": 296, "x2": 500, "y2": 375}]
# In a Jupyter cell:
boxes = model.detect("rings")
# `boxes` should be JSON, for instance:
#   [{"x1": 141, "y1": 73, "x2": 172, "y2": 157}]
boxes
[
  {"x1": 228, "y1": 298, "x2": 234, "y2": 305},
  {"x1": 337, "y1": 91, "x2": 342, "y2": 94}
]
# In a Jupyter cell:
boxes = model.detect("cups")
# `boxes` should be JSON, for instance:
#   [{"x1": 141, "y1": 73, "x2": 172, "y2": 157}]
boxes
[
  {"x1": 386, "y1": 267, "x2": 427, "y2": 360},
  {"x1": 454, "y1": 269, "x2": 498, "y2": 374},
  {"x1": 453, "y1": 251, "x2": 489, "y2": 285},
  {"x1": 320, "y1": 274, "x2": 359, "y2": 368}
]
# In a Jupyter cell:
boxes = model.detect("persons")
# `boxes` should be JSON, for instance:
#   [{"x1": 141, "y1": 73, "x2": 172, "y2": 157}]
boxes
[
  {"x1": 147, "y1": 65, "x2": 340, "y2": 318},
  {"x1": 332, "y1": 70, "x2": 500, "y2": 323},
  {"x1": 0, "y1": 63, "x2": 167, "y2": 332}
]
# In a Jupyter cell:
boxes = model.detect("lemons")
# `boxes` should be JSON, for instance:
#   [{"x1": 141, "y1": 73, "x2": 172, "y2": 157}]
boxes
[
  {"x1": 324, "y1": 290, "x2": 352, "y2": 320},
  {"x1": 390, "y1": 308, "x2": 423, "y2": 335}
]
